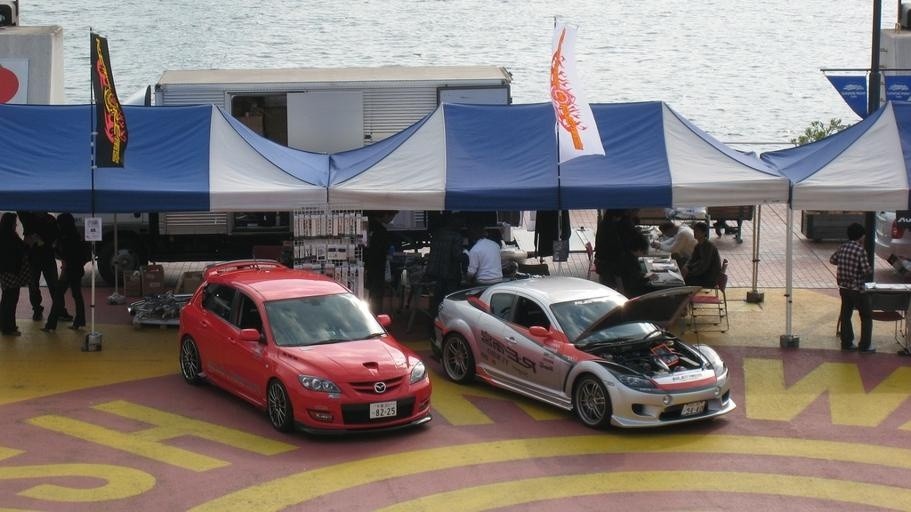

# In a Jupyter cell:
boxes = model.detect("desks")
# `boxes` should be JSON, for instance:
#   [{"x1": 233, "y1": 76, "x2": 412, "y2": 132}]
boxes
[{"x1": 845, "y1": 283, "x2": 911, "y2": 354}]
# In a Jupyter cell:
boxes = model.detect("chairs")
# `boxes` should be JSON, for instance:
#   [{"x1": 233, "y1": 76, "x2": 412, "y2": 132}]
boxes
[{"x1": 384, "y1": 220, "x2": 729, "y2": 334}]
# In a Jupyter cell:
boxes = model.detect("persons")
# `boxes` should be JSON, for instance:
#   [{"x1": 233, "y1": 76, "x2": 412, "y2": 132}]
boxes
[
  {"x1": 829, "y1": 222, "x2": 878, "y2": 354},
  {"x1": 593, "y1": 209, "x2": 723, "y2": 318},
  {"x1": 361, "y1": 210, "x2": 404, "y2": 314},
  {"x1": 459, "y1": 228, "x2": 505, "y2": 287},
  {"x1": 1, "y1": 210, "x2": 86, "y2": 337}
]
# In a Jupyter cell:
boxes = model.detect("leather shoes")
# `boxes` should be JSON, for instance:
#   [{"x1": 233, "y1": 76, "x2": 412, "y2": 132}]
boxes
[
  {"x1": 2, "y1": 330, "x2": 20, "y2": 336},
  {"x1": 67, "y1": 323, "x2": 83, "y2": 331},
  {"x1": 39, "y1": 327, "x2": 54, "y2": 333}
]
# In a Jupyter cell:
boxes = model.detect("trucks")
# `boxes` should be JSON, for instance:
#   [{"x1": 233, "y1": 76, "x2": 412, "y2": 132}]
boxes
[{"x1": 57, "y1": 67, "x2": 526, "y2": 287}]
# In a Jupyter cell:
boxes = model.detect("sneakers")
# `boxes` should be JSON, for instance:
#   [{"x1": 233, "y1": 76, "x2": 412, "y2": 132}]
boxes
[
  {"x1": 859, "y1": 346, "x2": 875, "y2": 354},
  {"x1": 59, "y1": 314, "x2": 73, "y2": 321},
  {"x1": 841, "y1": 345, "x2": 857, "y2": 351},
  {"x1": 32, "y1": 312, "x2": 42, "y2": 321}
]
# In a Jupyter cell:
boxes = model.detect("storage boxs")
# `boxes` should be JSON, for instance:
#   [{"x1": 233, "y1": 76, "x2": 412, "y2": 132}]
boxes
[
  {"x1": 123, "y1": 270, "x2": 139, "y2": 297},
  {"x1": 139, "y1": 265, "x2": 164, "y2": 296},
  {"x1": 183, "y1": 271, "x2": 201, "y2": 293}
]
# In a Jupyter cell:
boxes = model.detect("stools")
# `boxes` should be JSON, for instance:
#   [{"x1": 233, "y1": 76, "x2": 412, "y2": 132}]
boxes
[{"x1": 869, "y1": 310, "x2": 907, "y2": 348}]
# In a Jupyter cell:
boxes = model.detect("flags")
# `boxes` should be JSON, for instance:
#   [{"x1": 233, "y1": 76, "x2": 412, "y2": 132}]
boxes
[
  {"x1": 549, "y1": 19, "x2": 607, "y2": 164},
  {"x1": 88, "y1": 31, "x2": 129, "y2": 168}
]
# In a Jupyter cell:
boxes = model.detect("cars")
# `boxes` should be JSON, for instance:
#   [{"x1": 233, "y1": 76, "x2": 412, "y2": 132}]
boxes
[
  {"x1": 179, "y1": 259, "x2": 433, "y2": 436},
  {"x1": 873, "y1": 212, "x2": 911, "y2": 263},
  {"x1": 431, "y1": 275, "x2": 738, "y2": 429}
]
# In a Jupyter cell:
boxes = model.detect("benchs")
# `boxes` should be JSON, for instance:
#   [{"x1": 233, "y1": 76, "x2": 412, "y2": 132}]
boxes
[{"x1": 629, "y1": 206, "x2": 753, "y2": 244}]
